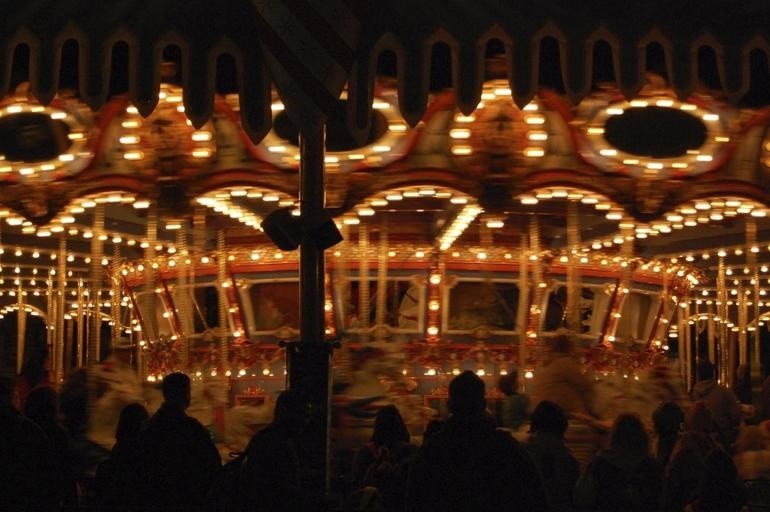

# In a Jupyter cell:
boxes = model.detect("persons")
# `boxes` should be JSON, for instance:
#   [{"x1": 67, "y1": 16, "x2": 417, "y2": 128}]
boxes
[{"x1": 1, "y1": 331, "x2": 769, "y2": 507}]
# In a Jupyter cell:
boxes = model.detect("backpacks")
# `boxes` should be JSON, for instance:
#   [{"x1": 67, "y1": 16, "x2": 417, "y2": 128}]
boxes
[{"x1": 345, "y1": 442, "x2": 416, "y2": 512}]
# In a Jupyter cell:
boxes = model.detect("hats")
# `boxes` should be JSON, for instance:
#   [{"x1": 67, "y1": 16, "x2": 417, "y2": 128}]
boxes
[{"x1": 448, "y1": 369, "x2": 486, "y2": 411}]
